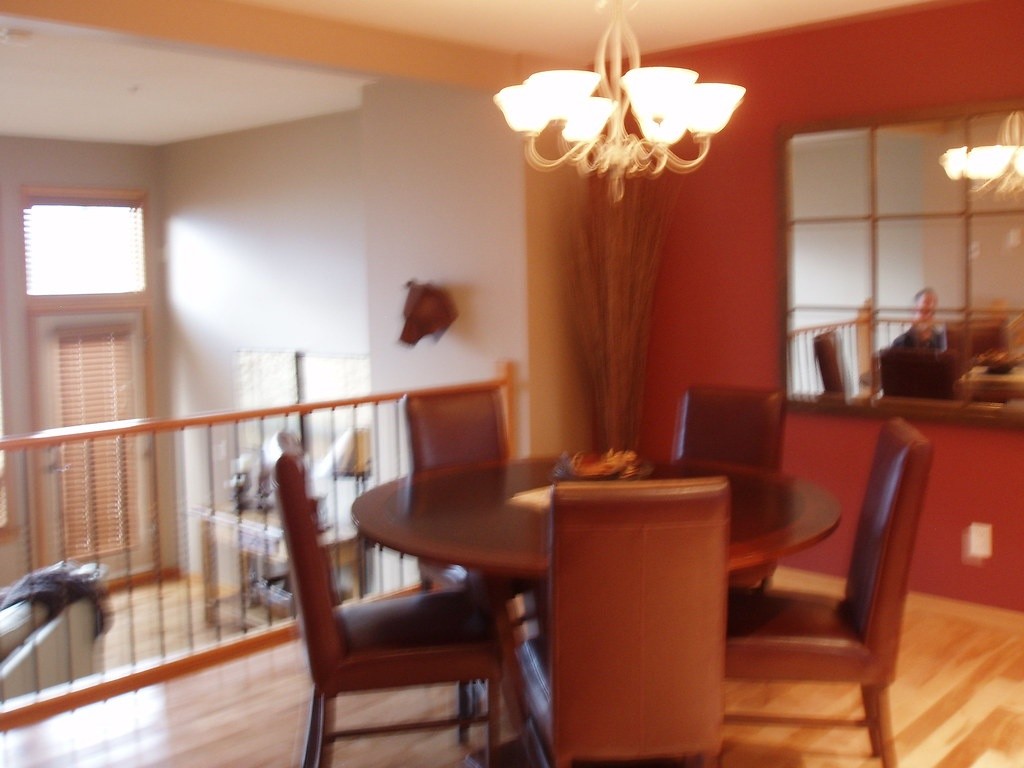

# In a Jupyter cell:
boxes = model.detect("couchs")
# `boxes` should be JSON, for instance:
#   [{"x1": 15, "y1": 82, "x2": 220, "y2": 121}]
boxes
[{"x1": 0, "y1": 562, "x2": 114, "y2": 705}]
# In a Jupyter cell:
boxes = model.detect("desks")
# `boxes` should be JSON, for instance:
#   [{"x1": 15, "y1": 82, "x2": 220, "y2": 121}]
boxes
[
  {"x1": 955, "y1": 366, "x2": 1024, "y2": 403},
  {"x1": 198, "y1": 502, "x2": 367, "y2": 627},
  {"x1": 353, "y1": 461, "x2": 845, "y2": 768}
]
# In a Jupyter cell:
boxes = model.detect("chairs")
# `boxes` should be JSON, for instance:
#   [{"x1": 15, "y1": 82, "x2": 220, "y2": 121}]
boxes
[
  {"x1": 673, "y1": 385, "x2": 786, "y2": 596},
  {"x1": 277, "y1": 456, "x2": 505, "y2": 767},
  {"x1": 515, "y1": 475, "x2": 729, "y2": 768},
  {"x1": 945, "y1": 316, "x2": 1005, "y2": 359},
  {"x1": 404, "y1": 384, "x2": 510, "y2": 589},
  {"x1": 814, "y1": 332, "x2": 847, "y2": 395},
  {"x1": 726, "y1": 418, "x2": 934, "y2": 768},
  {"x1": 880, "y1": 347, "x2": 960, "y2": 401}
]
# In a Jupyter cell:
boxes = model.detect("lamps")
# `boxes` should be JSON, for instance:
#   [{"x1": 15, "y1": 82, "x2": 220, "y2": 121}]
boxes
[
  {"x1": 333, "y1": 428, "x2": 370, "y2": 500},
  {"x1": 493, "y1": 0, "x2": 746, "y2": 205},
  {"x1": 939, "y1": 111, "x2": 1024, "y2": 203}
]
